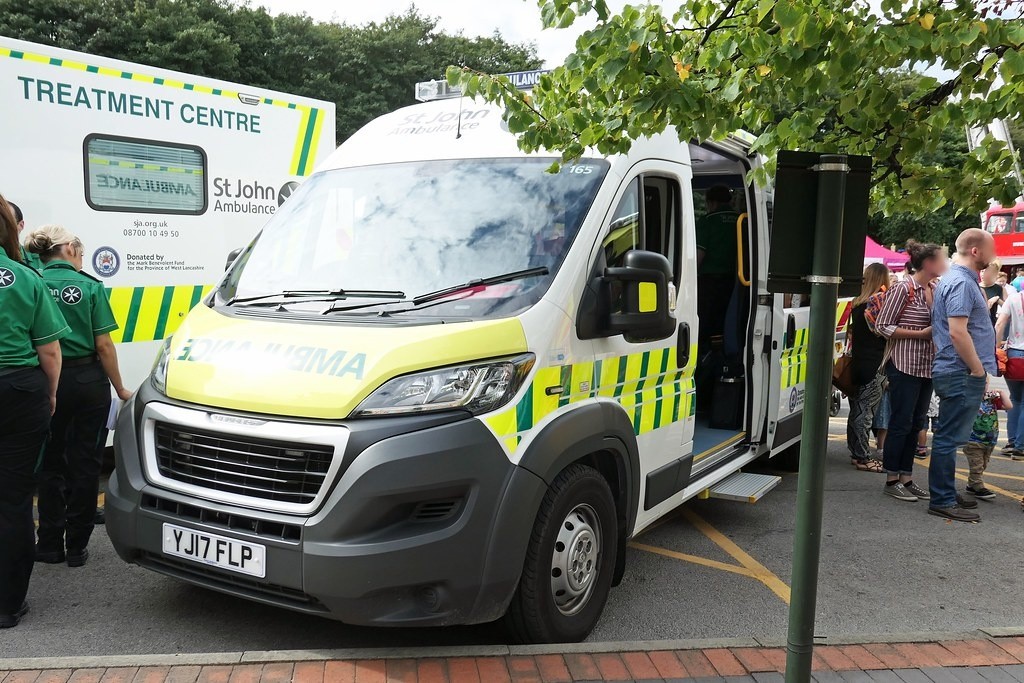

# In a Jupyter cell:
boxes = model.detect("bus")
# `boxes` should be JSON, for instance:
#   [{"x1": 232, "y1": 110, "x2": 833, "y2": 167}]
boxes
[{"x1": 977, "y1": 202, "x2": 1024, "y2": 286}]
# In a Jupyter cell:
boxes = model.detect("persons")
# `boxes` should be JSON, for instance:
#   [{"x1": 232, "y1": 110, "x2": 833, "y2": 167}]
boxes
[
  {"x1": 872, "y1": 387, "x2": 891, "y2": 462},
  {"x1": 865, "y1": 239, "x2": 945, "y2": 502},
  {"x1": 695, "y1": 182, "x2": 747, "y2": 356},
  {"x1": 930, "y1": 228, "x2": 995, "y2": 521},
  {"x1": 4, "y1": 198, "x2": 109, "y2": 526},
  {"x1": 0, "y1": 194, "x2": 71, "y2": 624},
  {"x1": 962, "y1": 375, "x2": 1012, "y2": 500},
  {"x1": 883, "y1": 214, "x2": 1023, "y2": 373},
  {"x1": 995, "y1": 288, "x2": 1024, "y2": 460},
  {"x1": 914, "y1": 386, "x2": 941, "y2": 458},
  {"x1": 24, "y1": 221, "x2": 133, "y2": 566},
  {"x1": 832, "y1": 263, "x2": 890, "y2": 472}
]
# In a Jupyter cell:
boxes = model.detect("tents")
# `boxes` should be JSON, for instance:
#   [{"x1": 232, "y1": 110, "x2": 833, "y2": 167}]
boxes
[{"x1": 864, "y1": 235, "x2": 910, "y2": 271}]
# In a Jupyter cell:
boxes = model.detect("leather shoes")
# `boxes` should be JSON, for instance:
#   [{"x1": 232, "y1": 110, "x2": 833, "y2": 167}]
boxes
[
  {"x1": 0, "y1": 597, "x2": 31, "y2": 629},
  {"x1": 32, "y1": 542, "x2": 89, "y2": 567}
]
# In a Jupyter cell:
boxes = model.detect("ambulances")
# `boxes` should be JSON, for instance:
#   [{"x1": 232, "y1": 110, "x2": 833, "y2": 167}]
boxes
[
  {"x1": 0, "y1": 34, "x2": 338, "y2": 453},
  {"x1": 95, "y1": 67, "x2": 814, "y2": 647}
]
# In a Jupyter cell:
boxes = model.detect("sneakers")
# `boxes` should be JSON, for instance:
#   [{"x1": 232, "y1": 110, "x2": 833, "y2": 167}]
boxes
[{"x1": 850, "y1": 442, "x2": 1024, "y2": 522}]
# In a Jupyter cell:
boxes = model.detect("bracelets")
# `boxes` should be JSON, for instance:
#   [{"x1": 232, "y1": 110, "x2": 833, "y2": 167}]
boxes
[{"x1": 116, "y1": 387, "x2": 124, "y2": 391}]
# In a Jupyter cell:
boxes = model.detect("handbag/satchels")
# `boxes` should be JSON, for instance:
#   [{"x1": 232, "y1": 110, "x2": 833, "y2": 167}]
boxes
[{"x1": 832, "y1": 352, "x2": 859, "y2": 397}]
